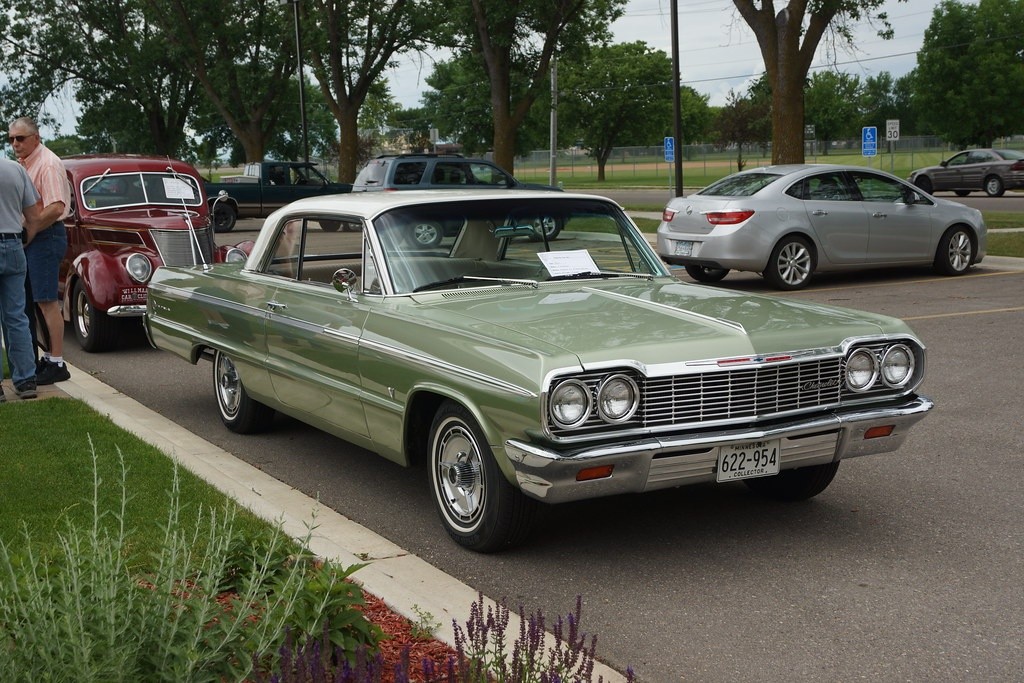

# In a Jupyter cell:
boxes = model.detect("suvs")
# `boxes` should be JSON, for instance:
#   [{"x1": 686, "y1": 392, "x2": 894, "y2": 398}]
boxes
[{"x1": 350, "y1": 153, "x2": 571, "y2": 248}]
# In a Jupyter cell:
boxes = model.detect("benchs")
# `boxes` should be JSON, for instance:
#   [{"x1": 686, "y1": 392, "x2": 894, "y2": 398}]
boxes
[{"x1": 268, "y1": 256, "x2": 492, "y2": 294}]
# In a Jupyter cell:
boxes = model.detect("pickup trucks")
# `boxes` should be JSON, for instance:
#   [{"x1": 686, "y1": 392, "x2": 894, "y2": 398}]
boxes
[{"x1": 199, "y1": 161, "x2": 352, "y2": 234}]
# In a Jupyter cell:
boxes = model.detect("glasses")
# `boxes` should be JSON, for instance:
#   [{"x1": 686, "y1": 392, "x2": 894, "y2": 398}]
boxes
[{"x1": 7, "y1": 133, "x2": 35, "y2": 144}]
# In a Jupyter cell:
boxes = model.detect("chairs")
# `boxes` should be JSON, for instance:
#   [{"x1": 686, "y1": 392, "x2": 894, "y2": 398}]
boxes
[
  {"x1": 810, "y1": 179, "x2": 838, "y2": 201},
  {"x1": 449, "y1": 168, "x2": 464, "y2": 184},
  {"x1": 433, "y1": 168, "x2": 445, "y2": 183}
]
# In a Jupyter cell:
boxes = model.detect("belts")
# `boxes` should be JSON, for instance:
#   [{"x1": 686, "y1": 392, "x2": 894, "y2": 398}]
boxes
[{"x1": 0, "y1": 233, "x2": 22, "y2": 240}]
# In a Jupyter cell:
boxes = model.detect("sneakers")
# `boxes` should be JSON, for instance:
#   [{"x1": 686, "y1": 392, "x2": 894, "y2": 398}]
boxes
[
  {"x1": 35, "y1": 357, "x2": 70, "y2": 385},
  {"x1": 0, "y1": 384, "x2": 6, "y2": 401},
  {"x1": 15, "y1": 381, "x2": 37, "y2": 399}
]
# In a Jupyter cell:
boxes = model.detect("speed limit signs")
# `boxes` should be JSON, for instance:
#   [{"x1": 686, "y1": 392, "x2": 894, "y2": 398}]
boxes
[{"x1": 886, "y1": 120, "x2": 899, "y2": 140}]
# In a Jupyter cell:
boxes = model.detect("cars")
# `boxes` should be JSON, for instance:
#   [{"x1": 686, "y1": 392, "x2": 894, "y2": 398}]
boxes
[
  {"x1": 907, "y1": 148, "x2": 1024, "y2": 197},
  {"x1": 143, "y1": 186, "x2": 934, "y2": 553},
  {"x1": 655, "y1": 163, "x2": 988, "y2": 290},
  {"x1": 55, "y1": 154, "x2": 247, "y2": 355}
]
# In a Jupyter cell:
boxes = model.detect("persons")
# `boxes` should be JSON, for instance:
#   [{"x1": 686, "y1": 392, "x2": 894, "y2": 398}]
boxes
[
  {"x1": 9, "y1": 117, "x2": 71, "y2": 386},
  {"x1": 0, "y1": 157, "x2": 42, "y2": 402}
]
